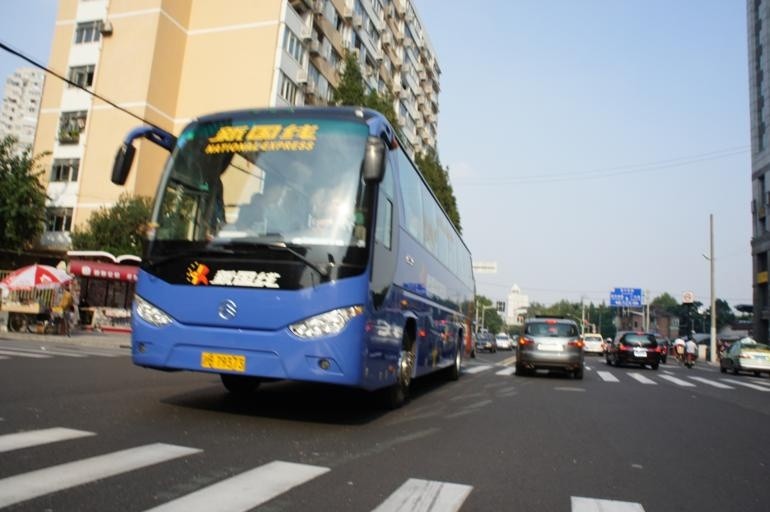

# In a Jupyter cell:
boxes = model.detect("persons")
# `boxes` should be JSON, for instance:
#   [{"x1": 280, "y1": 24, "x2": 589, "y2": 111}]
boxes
[
  {"x1": 672, "y1": 336, "x2": 686, "y2": 359},
  {"x1": 683, "y1": 335, "x2": 698, "y2": 366},
  {"x1": 64, "y1": 296, "x2": 78, "y2": 337}
]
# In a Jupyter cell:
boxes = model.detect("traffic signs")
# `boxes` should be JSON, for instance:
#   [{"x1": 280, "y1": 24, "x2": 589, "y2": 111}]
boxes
[{"x1": 609, "y1": 287, "x2": 642, "y2": 309}]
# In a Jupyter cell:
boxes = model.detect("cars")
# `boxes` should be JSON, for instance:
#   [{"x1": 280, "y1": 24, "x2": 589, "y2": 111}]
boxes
[{"x1": 473, "y1": 313, "x2": 770, "y2": 380}]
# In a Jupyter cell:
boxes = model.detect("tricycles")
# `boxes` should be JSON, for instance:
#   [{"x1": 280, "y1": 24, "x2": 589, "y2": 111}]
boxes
[{"x1": 7, "y1": 306, "x2": 74, "y2": 340}]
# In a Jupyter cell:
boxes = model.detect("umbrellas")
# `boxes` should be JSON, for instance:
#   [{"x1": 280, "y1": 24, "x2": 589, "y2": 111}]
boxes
[{"x1": 1, "y1": 262, "x2": 74, "y2": 302}]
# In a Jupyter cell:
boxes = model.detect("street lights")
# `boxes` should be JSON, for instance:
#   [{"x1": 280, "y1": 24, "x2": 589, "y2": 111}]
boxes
[{"x1": 701, "y1": 253, "x2": 718, "y2": 365}]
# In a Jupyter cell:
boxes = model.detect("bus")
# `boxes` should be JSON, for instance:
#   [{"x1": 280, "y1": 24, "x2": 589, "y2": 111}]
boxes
[{"x1": 105, "y1": 101, "x2": 478, "y2": 413}]
